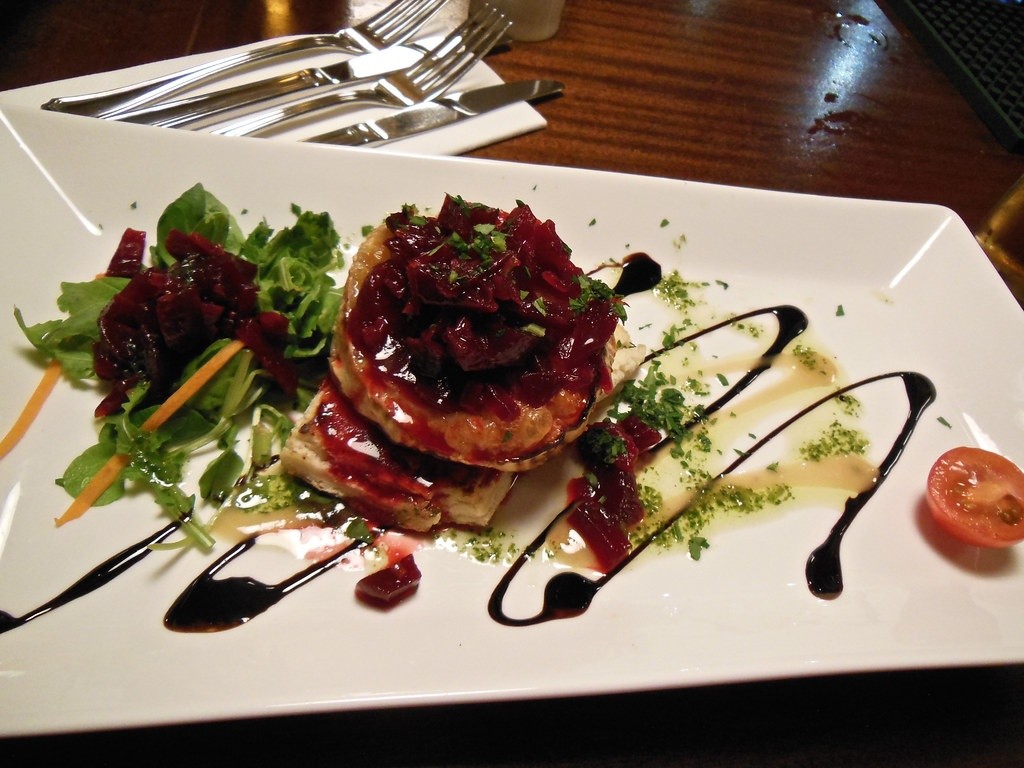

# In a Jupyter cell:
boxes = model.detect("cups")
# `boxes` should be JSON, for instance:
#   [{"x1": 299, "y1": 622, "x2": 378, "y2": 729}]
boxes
[{"x1": 469, "y1": 0, "x2": 565, "y2": 42}]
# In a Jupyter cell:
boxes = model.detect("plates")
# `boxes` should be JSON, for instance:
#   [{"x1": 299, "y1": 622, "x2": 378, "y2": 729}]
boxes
[{"x1": 0, "y1": 100, "x2": 1024, "y2": 736}]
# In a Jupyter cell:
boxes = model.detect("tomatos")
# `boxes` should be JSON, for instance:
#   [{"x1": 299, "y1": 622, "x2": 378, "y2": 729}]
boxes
[{"x1": 926, "y1": 447, "x2": 1024, "y2": 547}]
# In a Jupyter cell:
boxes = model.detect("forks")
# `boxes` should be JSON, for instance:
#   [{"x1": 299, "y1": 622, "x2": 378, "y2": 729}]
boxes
[
  {"x1": 39, "y1": 0, "x2": 452, "y2": 125},
  {"x1": 182, "y1": 4, "x2": 512, "y2": 138}
]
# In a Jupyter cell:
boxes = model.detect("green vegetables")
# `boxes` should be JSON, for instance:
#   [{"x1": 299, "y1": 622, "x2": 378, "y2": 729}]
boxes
[{"x1": 14, "y1": 182, "x2": 348, "y2": 550}]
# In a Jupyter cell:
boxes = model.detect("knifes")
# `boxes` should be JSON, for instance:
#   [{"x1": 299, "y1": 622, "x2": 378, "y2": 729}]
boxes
[
  {"x1": 291, "y1": 77, "x2": 567, "y2": 146},
  {"x1": 103, "y1": 30, "x2": 513, "y2": 129}
]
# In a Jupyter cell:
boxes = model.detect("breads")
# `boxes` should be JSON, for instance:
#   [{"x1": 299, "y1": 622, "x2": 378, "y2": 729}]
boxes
[{"x1": 279, "y1": 212, "x2": 614, "y2": 528}]
